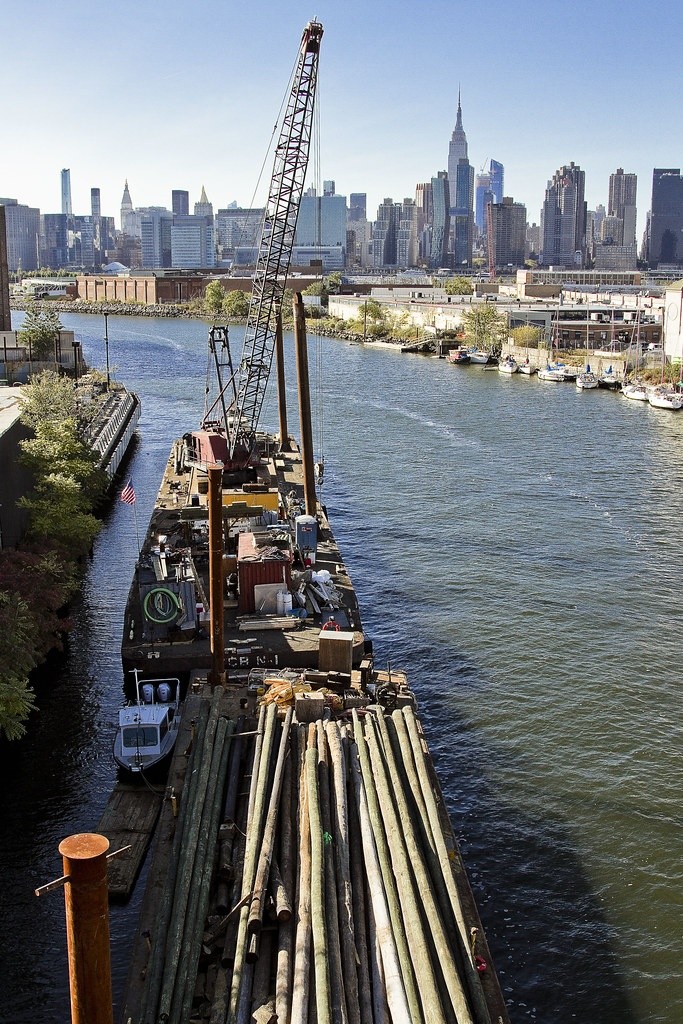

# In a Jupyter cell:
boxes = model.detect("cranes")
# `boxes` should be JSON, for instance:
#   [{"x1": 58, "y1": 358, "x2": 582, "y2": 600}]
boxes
[{"x1": 183, "y1": 15, "x2": 330, "y2": 479}]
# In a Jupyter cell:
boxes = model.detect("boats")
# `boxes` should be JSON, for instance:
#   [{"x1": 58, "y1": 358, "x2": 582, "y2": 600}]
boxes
[{"x1": 112, "y1": 667, "x2": 182, "y2": 785}]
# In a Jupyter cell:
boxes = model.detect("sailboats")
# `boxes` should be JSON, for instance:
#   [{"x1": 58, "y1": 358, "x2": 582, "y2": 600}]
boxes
[{"x1": 447, "y1": 289, "x2": 682, "y2": 410}]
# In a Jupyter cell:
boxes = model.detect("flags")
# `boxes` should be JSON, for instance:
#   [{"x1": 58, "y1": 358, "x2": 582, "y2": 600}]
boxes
[{"x1": 120, "y1": 478, "x2": 136, "y2": 505}]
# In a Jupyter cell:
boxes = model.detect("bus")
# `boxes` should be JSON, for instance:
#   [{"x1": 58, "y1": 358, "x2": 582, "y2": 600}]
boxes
[{"x1": 35, "y1": 284, "x2": 67, "y2": 298}]
